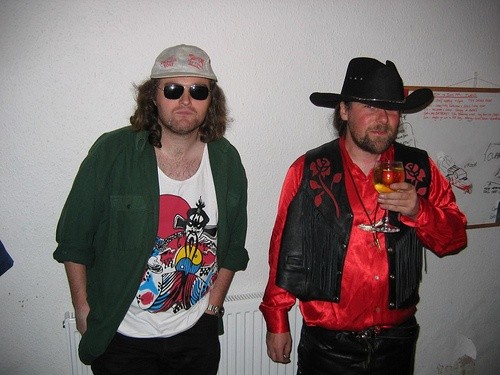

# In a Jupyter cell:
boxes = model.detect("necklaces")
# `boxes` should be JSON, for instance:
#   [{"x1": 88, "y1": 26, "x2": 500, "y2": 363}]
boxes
[{"x1": 344, "y1": 159, "x2": 400, "y2": 248}]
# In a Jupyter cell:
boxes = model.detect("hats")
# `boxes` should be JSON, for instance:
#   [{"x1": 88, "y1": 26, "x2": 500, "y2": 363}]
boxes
[
  {"x1": 150, "y1": 44, "x2": 218, "y2": 82},
  {"x1": 309, "y1": 57, "x2": 435, "y2": 115}
]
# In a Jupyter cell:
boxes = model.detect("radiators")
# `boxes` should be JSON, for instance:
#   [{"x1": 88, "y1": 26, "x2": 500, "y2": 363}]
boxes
[{"x1": 63, "y1": 294, "x2": 303, "y2": 375}]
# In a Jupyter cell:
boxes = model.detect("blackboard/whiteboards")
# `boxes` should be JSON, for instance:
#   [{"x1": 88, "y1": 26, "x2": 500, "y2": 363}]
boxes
[{"x1": 395, "y1": 86, "x2": 500, "y2": 229}]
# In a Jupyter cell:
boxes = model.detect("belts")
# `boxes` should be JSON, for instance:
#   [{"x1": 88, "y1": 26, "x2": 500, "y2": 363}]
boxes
[{"x1": 319, "y1": 319, "x2": 421, "y2": 342}]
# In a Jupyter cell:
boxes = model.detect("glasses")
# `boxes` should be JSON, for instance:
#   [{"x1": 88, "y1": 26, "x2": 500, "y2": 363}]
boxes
[{"x1": 157, "y1": 82, "x2": 212, "y2": 101}]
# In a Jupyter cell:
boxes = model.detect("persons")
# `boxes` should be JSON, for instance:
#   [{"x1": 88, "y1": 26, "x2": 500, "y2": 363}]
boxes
[
  {"x1": 257, "y1": 56, "x2": 468, "y2": 375},
  {"x1": 53, "y1": 45, "x2": 252, "y2": 375}
]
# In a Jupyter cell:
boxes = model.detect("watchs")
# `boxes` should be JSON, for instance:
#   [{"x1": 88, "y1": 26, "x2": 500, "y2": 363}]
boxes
[{"x1": 207, "y1": 303, "x2": 225, "y2": 318}]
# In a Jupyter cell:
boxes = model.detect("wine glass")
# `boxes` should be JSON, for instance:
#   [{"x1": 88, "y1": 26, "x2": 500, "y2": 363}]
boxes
[{"x1": 371, "y1": 161, "x2": 405, "y2": 233}]
